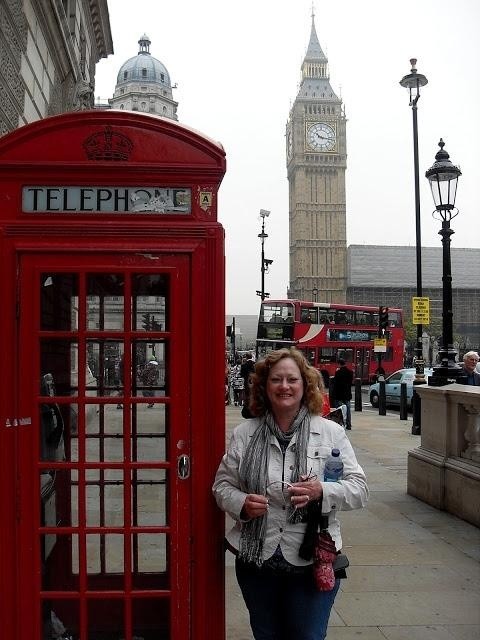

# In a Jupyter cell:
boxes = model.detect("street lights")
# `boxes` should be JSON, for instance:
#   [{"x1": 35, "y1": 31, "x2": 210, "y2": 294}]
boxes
[{"x1": 425, "y1": 137, "x2": 462, "y2": 373}]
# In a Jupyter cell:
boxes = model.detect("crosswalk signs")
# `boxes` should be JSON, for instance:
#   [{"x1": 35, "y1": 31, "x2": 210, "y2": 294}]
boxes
[{"x1": 379, "y1": 326, "x2": 387, "y2": 335}]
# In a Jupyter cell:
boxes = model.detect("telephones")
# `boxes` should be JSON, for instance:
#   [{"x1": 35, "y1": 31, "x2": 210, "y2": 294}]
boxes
[{"x1": 41, "y1": 371, "x2": 68, "y2": 451}]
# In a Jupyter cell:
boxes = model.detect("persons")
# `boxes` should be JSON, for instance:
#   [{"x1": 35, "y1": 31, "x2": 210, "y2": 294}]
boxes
[
  {"x1": 211, "y1": 347, "x2": 370, "y2": 640},
  {"x1": 462, "y1": 350, "x2": 480, "y2": 385},
  {"x1": 335, "y1": 358, "x2": 354, "y2": 431},
  {"x1": 139, "y1": 354, "x2": 160, "y2": 408},
  {"x1": 226, "y1": 352, "x2": 256, "y2": 410},
  {"x1": 271, "y1": 313, "x2": 293, "y2": 325},
  {"x1": 304, "y1": 313, "x2": 371, "y2": 325},
  {"x1": 115, "y1": 353, "x2": 125, "y2": 409}
]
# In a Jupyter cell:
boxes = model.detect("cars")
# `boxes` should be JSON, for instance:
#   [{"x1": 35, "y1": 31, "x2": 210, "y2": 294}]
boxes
[
  {"x1": 66, "y1": 342, "x2": 99, "y2": 436},
  {"x1": 367, "y1": 366, "x2": 434, "y2": 409}
]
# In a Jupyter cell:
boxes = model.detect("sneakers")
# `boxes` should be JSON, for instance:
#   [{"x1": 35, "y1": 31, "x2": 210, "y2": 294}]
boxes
[{"x1": 346, "y1": 426, "x2": 351, "y2": 430}]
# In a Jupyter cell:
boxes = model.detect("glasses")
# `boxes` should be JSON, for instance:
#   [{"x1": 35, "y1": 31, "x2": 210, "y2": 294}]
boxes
[{"x1": 302, "y1": 468, "x2": 317, "y2": 482}]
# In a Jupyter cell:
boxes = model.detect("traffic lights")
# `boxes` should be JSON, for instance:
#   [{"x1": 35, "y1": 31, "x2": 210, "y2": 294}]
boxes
[
  {"x1": 141, "y1": 314, "x2": 151, "y2": 331},
  {"x1": 379, "y1": 305, "x2": 389, "y2": 327}
]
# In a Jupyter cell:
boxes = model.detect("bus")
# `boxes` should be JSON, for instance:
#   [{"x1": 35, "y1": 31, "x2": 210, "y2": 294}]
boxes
[{"x1": 254, "y1": 298, "x2": 406, "y2": 388}]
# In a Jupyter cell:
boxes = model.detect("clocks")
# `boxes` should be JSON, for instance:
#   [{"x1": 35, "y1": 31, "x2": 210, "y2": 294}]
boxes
[{"x1": 305, "y1": 121, "x2": 337, "y2": 153}]
